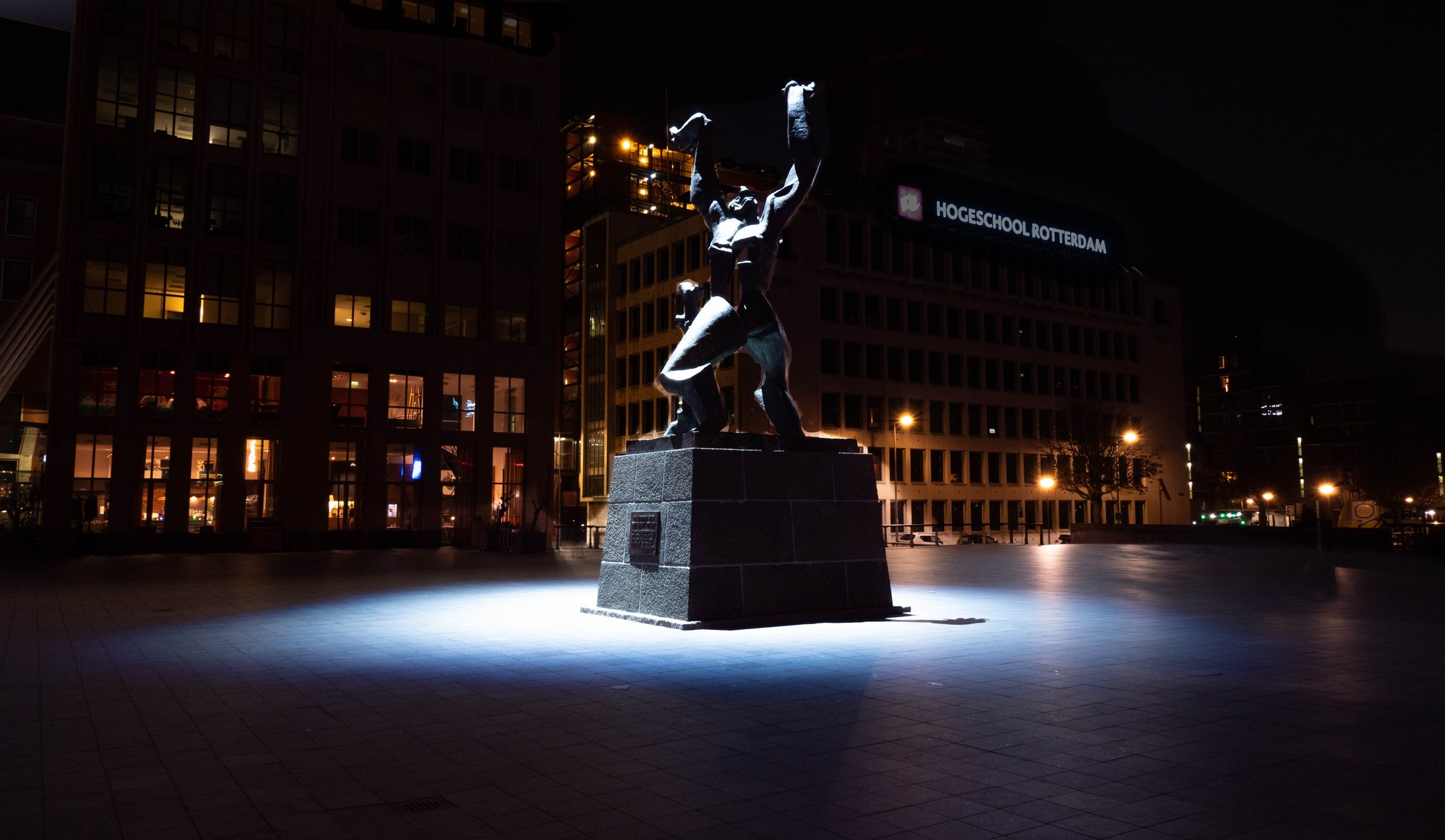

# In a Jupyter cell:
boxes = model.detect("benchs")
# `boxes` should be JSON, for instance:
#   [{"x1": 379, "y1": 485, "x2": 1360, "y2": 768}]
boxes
[
  {"x1": 337, "y1": 416, "x2": 365, "y2": 427},
  {"x1": 389, "y1": 418, "x2": 419, "y2": 429}
]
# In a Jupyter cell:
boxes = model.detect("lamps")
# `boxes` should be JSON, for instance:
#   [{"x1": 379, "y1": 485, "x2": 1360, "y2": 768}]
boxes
[
  {"x1": 246, "y1": 497, "x2": 250, "y2": 501},
  {"x1": 164, "y1": 452, "x2": 168, "y2": 459}
]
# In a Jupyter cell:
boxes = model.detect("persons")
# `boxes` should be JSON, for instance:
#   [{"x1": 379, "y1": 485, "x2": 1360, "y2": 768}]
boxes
[
  {"x1": 658, "y1": 79, "x2": 829, "y2": 448},
  {"x1": 671, "y1": 278, "x2": 703, "y2": 435}
]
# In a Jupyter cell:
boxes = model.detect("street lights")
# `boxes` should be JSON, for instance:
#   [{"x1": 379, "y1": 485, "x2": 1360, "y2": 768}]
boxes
[
  {"x1": 893, "y1": 412, "x2": 916, "y2": 543},
  {"x1": 1039, "y1": 477, "x2": 1056, "y2": 544}
]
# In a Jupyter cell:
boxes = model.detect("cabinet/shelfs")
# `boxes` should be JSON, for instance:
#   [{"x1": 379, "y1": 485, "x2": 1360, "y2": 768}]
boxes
[{"x1": 199, "y1": 474, "x2": 216, "y2": 479}]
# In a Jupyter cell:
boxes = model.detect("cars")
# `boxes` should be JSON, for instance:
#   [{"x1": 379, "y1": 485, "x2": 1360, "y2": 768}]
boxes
[{"x1": 956, "y1": 534, "x2": 1002, "y2": 544}]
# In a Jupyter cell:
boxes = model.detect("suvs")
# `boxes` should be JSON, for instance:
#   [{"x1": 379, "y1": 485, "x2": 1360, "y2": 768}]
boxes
[{"x1": 897, "y1": 533, "x2": 951, "y2": 547}]
